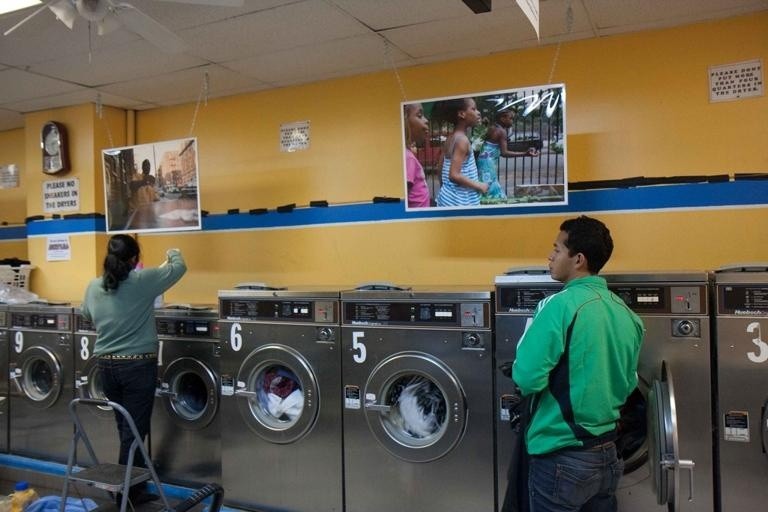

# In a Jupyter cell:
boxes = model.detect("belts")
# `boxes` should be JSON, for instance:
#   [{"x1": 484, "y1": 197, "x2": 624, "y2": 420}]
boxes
[{"x1": 98, "y1": 353, "x2": 157, "y2": 360}]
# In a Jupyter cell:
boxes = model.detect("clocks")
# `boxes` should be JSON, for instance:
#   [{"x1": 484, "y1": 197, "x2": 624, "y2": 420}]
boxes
[{"x1": 41, "y1": 121, "x2": 67, "y2": 173}]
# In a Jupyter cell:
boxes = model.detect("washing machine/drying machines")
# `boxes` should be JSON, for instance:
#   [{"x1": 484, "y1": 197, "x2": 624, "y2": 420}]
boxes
[
  {"x1": 214, "y1": 278, "x2": 498, "y2": 510},
  {"x1": 482, "y1": 269, "x2": 767, "y2": 508},
  {"x1": 4, "y1": 297, "x2": 222, "y2": 501}
]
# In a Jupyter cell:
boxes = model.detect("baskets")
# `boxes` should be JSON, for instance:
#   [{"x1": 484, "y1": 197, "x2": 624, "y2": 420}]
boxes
[{"x1": 0, "y1": 264, "x2": 37, "y2": 292}]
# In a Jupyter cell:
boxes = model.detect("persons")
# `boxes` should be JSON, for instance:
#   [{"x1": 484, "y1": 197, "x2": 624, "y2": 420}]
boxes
[
  {"x1": 428, "y1": 97, "x2": 492, "y2": 208},
  {"x1": 510, "y1": 213, "x2": 647, "y2": 511},
  {"x1": 128, "y1": 159, "x2": 163, "y2": 229},
  {"x1": 401, "y1": 102, "x2": 436, "y2": 208},
  {"x1": 474, "y1": 108, "x2": 542, "y2": 202},
  {"x1": 77, "y1": 230, "x2": 189, "y2": 510}
]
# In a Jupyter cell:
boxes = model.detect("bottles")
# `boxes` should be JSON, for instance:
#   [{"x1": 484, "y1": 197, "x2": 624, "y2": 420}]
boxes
[{"x1": 10, "y1": 480, "x2": 40, "y2": 512}]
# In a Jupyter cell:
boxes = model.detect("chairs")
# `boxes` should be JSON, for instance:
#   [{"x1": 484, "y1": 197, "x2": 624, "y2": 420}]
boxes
[{"x1": 60, "y1": 398, "x2": 224, "y2": 511}]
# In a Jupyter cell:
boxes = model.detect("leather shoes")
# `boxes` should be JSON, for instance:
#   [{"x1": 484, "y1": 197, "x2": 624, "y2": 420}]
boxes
[{"x1": 134, "y1": 491, "x2": 159, "y2": 505}]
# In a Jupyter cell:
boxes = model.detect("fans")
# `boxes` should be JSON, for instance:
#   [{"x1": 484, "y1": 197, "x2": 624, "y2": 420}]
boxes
[{"x1": 0, "y1": 1, "x2": 244, "y2": 57}]
